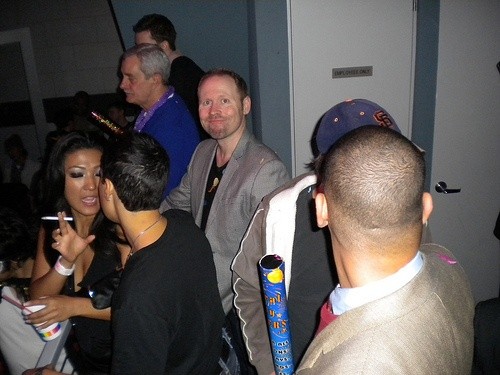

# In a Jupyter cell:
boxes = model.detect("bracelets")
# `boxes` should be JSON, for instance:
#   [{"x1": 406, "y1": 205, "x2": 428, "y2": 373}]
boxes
[{"x1": 54, "y1": 255, "x2": 75, "y2": 276}]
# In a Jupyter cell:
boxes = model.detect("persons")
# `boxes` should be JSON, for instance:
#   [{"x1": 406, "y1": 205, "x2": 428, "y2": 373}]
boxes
[
  {"x1": 0, "y1": 90, "x2": 134, "y2": 301},
  {"x1": 22, "y1": 131, "x2": 131, "y2": 375},
  {"x1": 133, "y1": 13, "x2": 212, "y2": 143},
  {"x1": 294, "y1": 125, "x2": 475, "y2": 375},
  {"x1": 119, "y1": 43, "x2": 199, "y2": 203},
  {"x1": 23, "y1": 133, "x2": 241, "y2": 375},
  {"x1": 160, "y1": 67, "x2": 290, "y2": 375},
  {"x1": 230, "y1": 98, "x2": 401, "y2": 375}
]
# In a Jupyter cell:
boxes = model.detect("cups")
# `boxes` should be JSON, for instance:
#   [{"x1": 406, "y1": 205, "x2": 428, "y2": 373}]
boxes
[{"x1": 22, "y1": 305, "x2": 62, "y2": 341}]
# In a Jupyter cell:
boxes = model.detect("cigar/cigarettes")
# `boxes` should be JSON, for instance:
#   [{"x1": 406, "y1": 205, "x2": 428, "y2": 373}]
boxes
[{"x1": 41, "y1": 216, "x2": 73, "y2": 221}]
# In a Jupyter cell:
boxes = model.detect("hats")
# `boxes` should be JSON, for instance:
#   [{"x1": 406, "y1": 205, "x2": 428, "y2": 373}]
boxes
[{"x1": 316, "y1": 99, "x2": 425, "y2": 156}]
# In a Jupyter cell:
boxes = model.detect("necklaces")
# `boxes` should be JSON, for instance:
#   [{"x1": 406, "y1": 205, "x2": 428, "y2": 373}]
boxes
[{"x1": 127, "y1": 215, "x2": 163, "y2": 260}]
